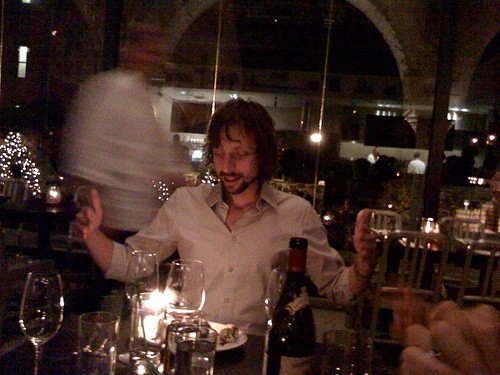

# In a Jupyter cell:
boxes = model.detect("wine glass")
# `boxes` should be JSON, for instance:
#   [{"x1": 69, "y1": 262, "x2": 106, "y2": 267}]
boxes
[
  {"x1": 20, "y1": 272, "x2": 63, "y2": 375},
  {"x1": 118, "y1": 249, "x2": 206, "y2": 375}
]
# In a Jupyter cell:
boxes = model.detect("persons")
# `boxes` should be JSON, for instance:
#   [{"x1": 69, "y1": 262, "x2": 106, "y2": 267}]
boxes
[
  {"x1": 399, "y1": 299, "x2": 500, "y2": 375},
  {"x1": 73, "y1": 99, "x2": 377, "y2": 336},
  {"x1": 173, "y1": 133, "x2": 188, "y2": 161},
  {"x1": 367, "y1": 147, "x2": 380, "y2": 164},
  {"x1": 407, "y1": 152, "x2": 426, "y2": 174},
  {"x1": 63, "y1": 26, "x2": 201, "y2": 314}
]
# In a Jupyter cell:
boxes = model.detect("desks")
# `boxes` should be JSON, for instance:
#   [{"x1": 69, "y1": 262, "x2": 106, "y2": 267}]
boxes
[
  {"x1": 378, "y1": 235, "x2": 499, "y2": 296},
  {"x1": 0, "y1": 316, "x2": 328, "y2": 375}
]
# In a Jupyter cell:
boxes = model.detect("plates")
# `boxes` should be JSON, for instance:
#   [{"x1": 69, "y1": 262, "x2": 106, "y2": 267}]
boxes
[{"x1": 189, "y1": 321, "x2": 247, "y2": 351}]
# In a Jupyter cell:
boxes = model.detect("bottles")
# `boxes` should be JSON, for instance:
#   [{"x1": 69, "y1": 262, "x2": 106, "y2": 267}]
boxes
[{"x1": 266, "y1": 236, "x2": 316, "y2": 375}]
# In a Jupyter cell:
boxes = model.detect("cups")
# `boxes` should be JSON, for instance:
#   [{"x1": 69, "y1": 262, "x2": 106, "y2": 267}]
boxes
[
  {"x1": 164, "y1": 322, "x2": 219, "y2": 375},
  {"x1": 128, "y1": 293, "x2": 166, "y2": 375},
  {"x1": 319, "y1": 330, "x2": 375, "y2": 375},
  {"x1": 264, "y1": 267, "x2": 287, "y2": 319},
  {"x1": 78, "y1": 311, "x2": 120, "y2": 375}
]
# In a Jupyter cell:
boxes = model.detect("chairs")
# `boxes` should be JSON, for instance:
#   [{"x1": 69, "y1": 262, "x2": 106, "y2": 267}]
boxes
[{"x1": 350, "y1": 210, "x2": 500, "y2": 375}]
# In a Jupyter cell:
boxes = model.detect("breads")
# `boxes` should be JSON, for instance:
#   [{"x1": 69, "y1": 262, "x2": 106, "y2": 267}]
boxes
[{"x1": 400, "y1": 300, "x2": 500, "y2": 375}]
